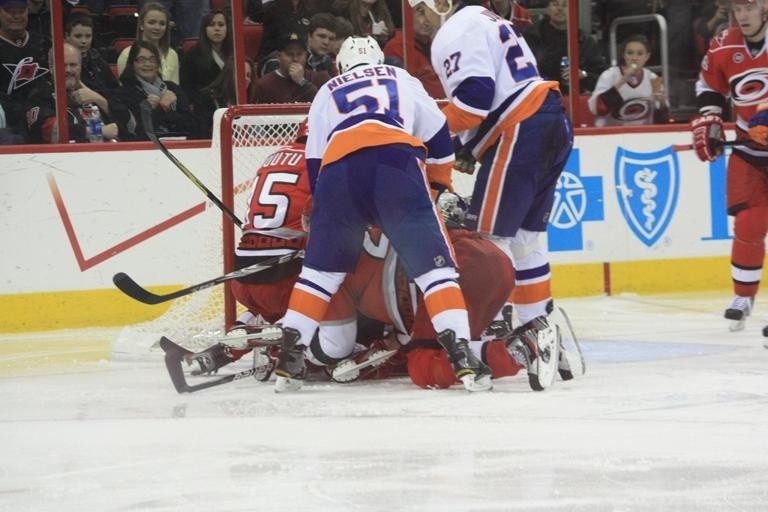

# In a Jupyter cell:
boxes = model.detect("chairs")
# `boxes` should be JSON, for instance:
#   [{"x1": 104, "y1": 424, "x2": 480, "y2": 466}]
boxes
[
  {"x1": 562, "y1": 94, "x2": 596, "y2": 128},
  {"x1": 63, "y1": 5, "x2": 265, "y2": 83}
]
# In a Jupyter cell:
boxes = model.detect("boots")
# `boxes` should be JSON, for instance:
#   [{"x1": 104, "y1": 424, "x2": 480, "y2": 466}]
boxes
[
  {"x1": 182, "y1": 342, "x2": 233, "y2": 375},
  {"x1": 324, "y1": 336, "x2": 401, "y2": 384},
  {"x1": 486, "y1": 298, "x2": 574, "y2": 392},
  {"x1": 220, "y1": 324, "x2": 311, "y2": 380},
  {"x1": 436, "y1": 327, "x2": 495, "y2": 381}
]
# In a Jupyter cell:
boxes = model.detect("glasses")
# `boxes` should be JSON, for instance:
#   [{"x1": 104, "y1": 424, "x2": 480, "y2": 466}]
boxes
[{"x1": 134, "y1": 55, "x2": 158, "y2": 63}]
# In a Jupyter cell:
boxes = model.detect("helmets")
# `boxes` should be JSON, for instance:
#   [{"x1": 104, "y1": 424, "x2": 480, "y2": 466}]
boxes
[
  {"x1": 335, "y1": 34, "x2": 385, "y2": 75},
  {"x1": 407, "y1": 0, "x2": 452, "y2": 11}
]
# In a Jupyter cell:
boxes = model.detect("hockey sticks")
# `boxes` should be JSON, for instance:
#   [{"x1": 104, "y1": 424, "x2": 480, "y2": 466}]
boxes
[
  {"x1": 140, "y1": 98, "x2": 246, "y2": 229},
  {"x1": 161, "y1": 337, "x2": 284, "y2": 395},
  {"x1": 113, "y1": 250, "x2": 306, "y2": 304}
]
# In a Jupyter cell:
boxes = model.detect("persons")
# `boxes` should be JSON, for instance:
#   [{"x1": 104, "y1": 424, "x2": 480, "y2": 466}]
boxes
[
  {"x1": 0, "y1": 1, "x2": 727, "y2": 145},
  {"x1": 691, "y1": 0, "x2": 767, "y2": 350},
  {"x1": 179, "y1": 0, "x2": 587, "y2": 392}
]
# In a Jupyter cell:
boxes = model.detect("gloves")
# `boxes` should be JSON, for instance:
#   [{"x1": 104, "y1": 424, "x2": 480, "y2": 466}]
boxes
[
  {"x1": 689, "y1": 113, "x2": 727, "y2": 162},
  {"x1": 748, "y1": 103, "x2": 768, "y2": 147},
  {"x1": 454, "y1": 148, "x2": 476, "y2": 174}
]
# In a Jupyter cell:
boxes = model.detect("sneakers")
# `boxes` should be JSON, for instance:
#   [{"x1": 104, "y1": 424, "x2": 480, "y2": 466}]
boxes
[{"x1": 725, "y1": 295, "x2": 755, "y2": 321}]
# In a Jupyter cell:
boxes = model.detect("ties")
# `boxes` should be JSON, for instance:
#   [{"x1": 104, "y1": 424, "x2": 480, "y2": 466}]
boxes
[{"x1": 276, "y1": 31, "x2": 307, "y2": 50}]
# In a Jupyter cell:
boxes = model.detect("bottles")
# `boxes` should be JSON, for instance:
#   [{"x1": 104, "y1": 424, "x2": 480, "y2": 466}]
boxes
[
  {"x1": 561, "y1": 56, "x2": 569, "y2": 86},
  {"x1": 88, "y1": 107, "x2": 102, "y2": 144},
  {"x1": 628, "y1": 63, "x2": 639, "y2": 86}
]
